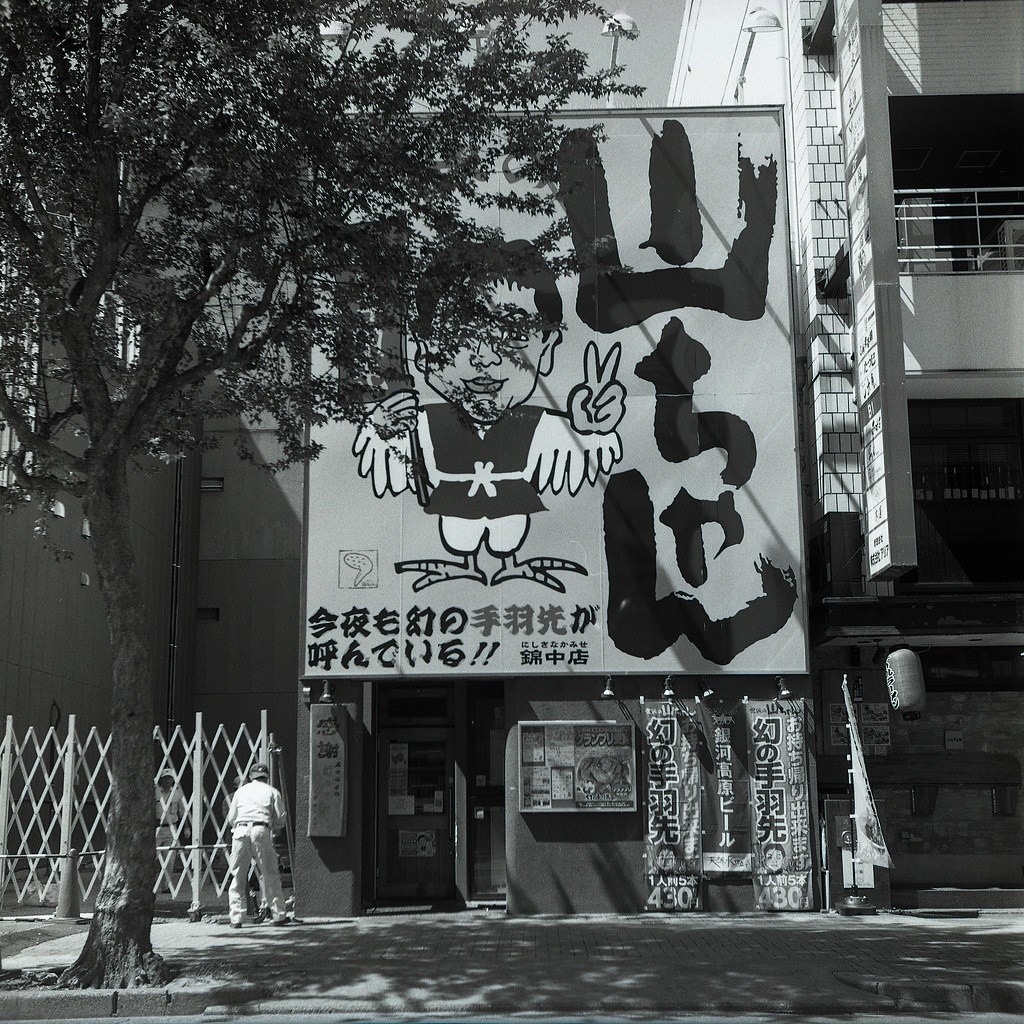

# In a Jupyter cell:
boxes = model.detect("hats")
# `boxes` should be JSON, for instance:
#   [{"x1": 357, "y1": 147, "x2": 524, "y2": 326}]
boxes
[
  {"x1": 249, "y1": 762, "x2": 270, "y2": 779},
  {"x1": 161, "y1": 769, "x2": 174, "y2": 777},
  {"x1": 233, "y1": 776, "x2": 249, "y2": 788}
]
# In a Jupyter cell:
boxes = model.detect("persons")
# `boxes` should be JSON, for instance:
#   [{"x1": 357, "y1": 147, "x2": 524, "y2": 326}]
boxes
[
  {"x1": 227, "y1": 763, "x2": 290, "y2": 928},
  {"x1": 152, "y1": 768, "x2": 189, "y2": 893},
  {"x1": 224, "y1": 775, "x2": 251, "y2": 843}
]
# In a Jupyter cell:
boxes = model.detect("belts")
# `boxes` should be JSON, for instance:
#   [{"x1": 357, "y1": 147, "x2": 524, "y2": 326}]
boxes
[
  {"x1": 239, "y1": 821, "x2": 269, "y2": 826},
  {"x1": 157, "y1": 823, "x2": 176, "y2": 827}
]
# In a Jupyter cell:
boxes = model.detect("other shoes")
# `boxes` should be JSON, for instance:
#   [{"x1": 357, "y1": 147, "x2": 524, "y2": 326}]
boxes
[
  {"x1": 229, "y1": 922, "x2": 242, "y2": 928},
  {"x1": 273, "y1": 917, "x2": 292, "y2": 927}
]
